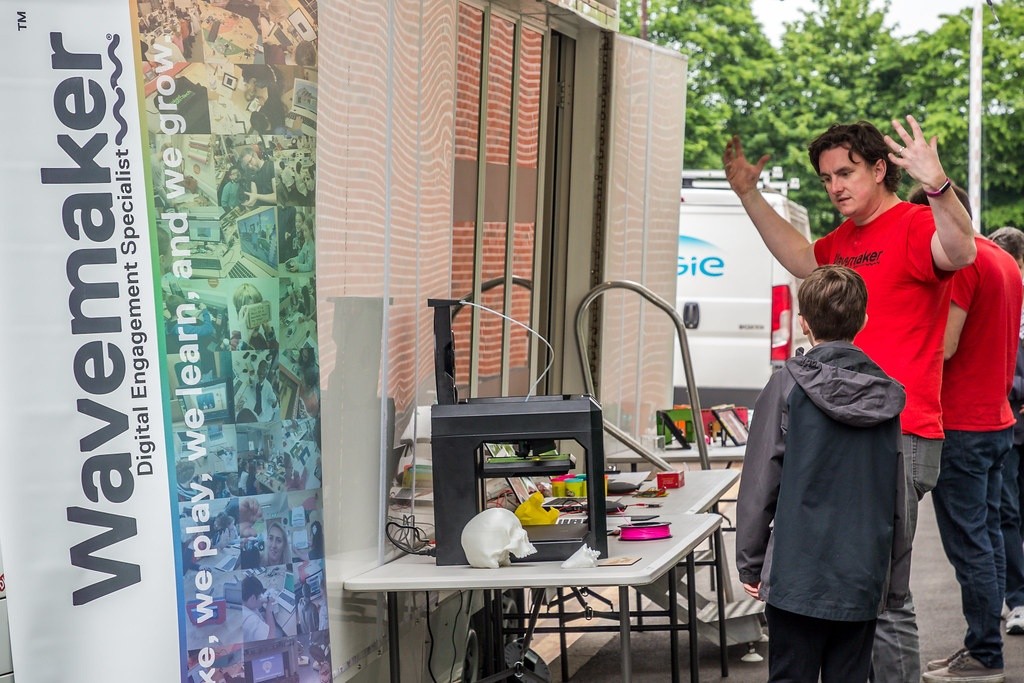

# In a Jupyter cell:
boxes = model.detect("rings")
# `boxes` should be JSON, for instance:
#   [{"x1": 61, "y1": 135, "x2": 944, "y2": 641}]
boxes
[{"x1": 898, "y1": 146, "x2": 905, "y2": 154}]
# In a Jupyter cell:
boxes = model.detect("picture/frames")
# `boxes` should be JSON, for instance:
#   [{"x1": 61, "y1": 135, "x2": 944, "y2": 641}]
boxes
[{"x1": 656, "y1": 405, "x2": 750, "y2": 447}]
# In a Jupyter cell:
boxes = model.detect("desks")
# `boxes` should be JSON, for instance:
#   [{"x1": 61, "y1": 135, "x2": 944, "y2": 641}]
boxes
[{"x1": 343, "y1": 435, "x2": 753, "y2": 683}]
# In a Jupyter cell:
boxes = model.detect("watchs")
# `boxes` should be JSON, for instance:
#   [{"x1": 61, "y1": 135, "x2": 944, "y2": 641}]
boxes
[{"x1": 922, "y1": 177, "x2": 952, "y2": 199}]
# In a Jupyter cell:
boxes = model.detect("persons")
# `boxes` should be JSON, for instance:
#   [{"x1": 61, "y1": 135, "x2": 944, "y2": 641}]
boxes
[
  {"x1": 906, "y1": 179, "x2": 1024, "y2": 683},
  {"x1": 129, "y1": 1, "x2": 334, "y2": 682},
  {"x1": 721, "y1": 112, "x2": 979, "y2": 683}
]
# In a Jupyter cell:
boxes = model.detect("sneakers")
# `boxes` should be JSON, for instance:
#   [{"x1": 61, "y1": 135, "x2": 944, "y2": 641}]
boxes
[
  {"x1": 1006, "y1": 605, "x2": 1024, "y2": 635},
  {"x1": 928, "y1": 647, "x2": 968, "y2": 670},
  {"x1": 921, "y1": 650, "x2": 1004, "y2": 683},
  {"x1": 1000, "y1": 596, "x2": 1011, "y2": 619}
]
